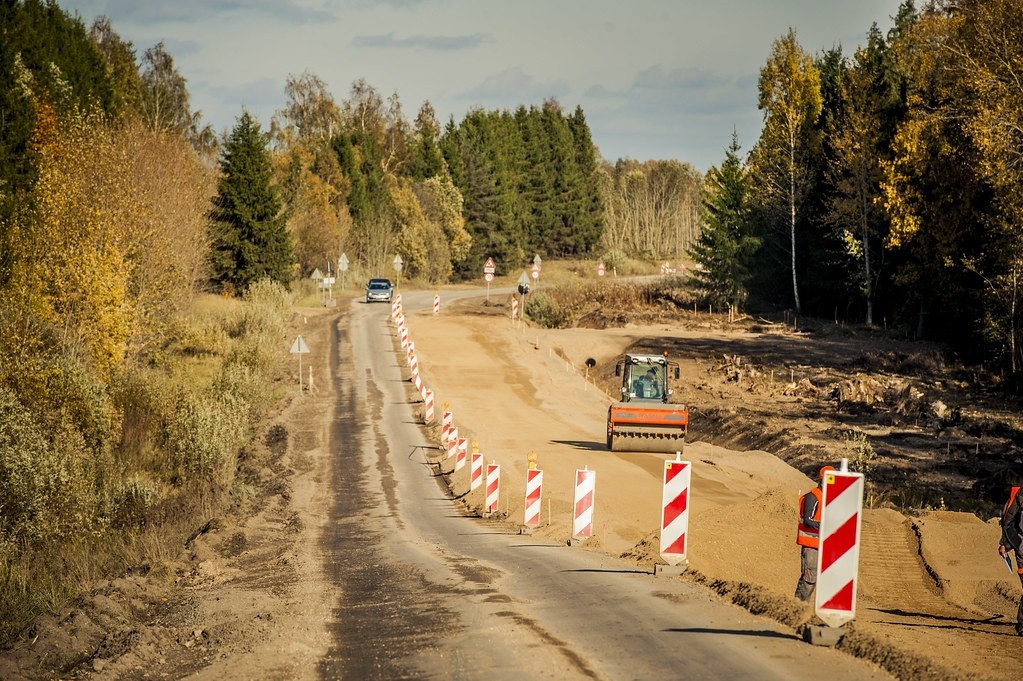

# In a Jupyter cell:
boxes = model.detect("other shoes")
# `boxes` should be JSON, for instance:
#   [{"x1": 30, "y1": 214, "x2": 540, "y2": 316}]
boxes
[{"x1": 1015, "y1": 624, "x2": 1023, "y2": 636}]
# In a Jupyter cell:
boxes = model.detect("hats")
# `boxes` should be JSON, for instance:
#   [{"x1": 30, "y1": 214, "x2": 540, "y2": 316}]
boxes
[
  {"x1": 821, "y1": 466, "x2": 836, "y2": 479},
  {"x1": 647, "y1": 370, "x2": 656, "y2": 374}
]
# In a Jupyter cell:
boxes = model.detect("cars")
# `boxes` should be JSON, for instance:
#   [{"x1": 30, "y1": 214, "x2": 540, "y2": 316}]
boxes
[{"x1": 365, "y1": 278, "x2": 395, "y2": 303}]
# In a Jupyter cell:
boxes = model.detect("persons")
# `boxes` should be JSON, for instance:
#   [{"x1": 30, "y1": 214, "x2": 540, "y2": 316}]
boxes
[
  {"x1": 794, "y1": 465, "x2": 837, "y2": 603},
  {"x1": 644, "y1": 366, "x2": 659, "y2": 381},
  {"x1": 998, "y1": 482, "x2": 1023, "y2": 638}
]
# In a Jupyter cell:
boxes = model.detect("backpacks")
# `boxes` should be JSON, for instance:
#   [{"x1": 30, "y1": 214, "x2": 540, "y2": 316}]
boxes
[{"x1": 999, "y1": 487, "x2": 1023, "y2": 550}]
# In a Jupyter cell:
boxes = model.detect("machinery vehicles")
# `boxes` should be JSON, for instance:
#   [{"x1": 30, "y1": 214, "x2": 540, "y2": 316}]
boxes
[{"x1": 606, "y1": 353, "x2": 689, "y2": 455}]
